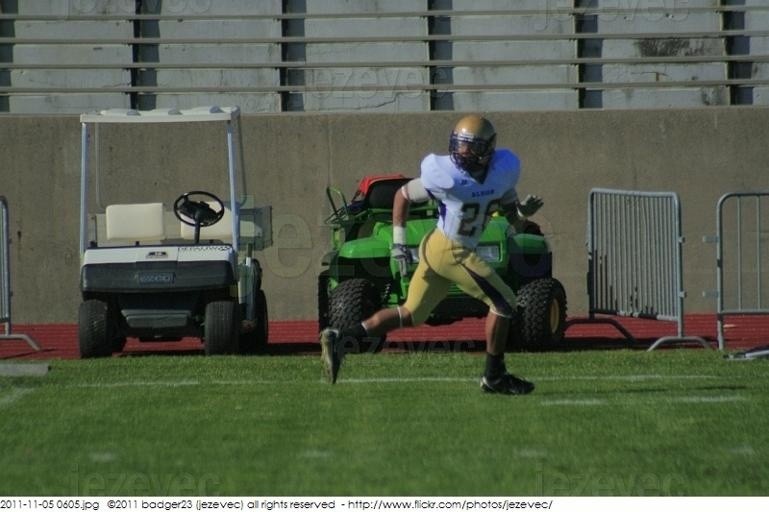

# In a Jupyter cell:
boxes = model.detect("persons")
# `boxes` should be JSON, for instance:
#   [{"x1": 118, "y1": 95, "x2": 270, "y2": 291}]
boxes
[{"x1": 318, "y1": 113, "x2": 544, "y2": 396}]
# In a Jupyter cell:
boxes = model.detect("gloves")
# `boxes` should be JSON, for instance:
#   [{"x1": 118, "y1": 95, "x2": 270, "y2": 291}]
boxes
[
  {"x1": 516, "y1": 193, "x2": 545, "y2": 222},
  {"x1": 390, "y1": 242, "x2": 414, "y2": 279}
]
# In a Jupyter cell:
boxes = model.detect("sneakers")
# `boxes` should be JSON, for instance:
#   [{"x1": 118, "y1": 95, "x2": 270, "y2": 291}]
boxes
[
  {"x1": 317, "y1": 327, "x2": 345, "y2": 386},
  {"x1": 479, "y1": 371, "x2": 535, "y2": 396}
]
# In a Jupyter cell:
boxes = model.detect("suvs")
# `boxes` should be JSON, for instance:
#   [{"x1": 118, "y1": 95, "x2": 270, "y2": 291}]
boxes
[{"x1": 314, "y1": 169, "x2": 571, "y2": 355}]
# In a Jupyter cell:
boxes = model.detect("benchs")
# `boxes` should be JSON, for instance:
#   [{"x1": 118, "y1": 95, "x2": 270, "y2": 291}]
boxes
[{"x1": 0, "y1": 5, "x2": 768, "y2": 96}]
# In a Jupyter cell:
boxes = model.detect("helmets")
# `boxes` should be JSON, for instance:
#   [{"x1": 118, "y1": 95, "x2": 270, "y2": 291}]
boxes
[{"x1": 448, "y1": 113, "x2": 498, "y2": 177}]
